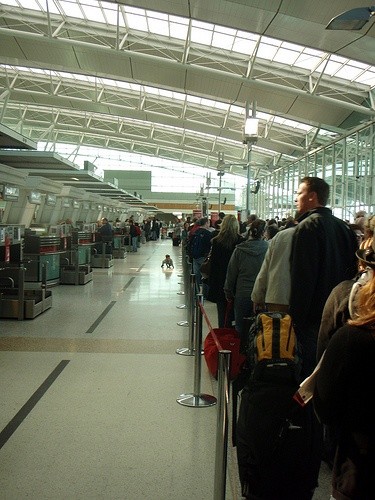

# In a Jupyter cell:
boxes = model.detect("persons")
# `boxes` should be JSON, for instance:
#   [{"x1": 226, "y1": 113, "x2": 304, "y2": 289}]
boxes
[
  {"x1": 206, "y1": 215, "x2": 246, "y2": 328},
  {"x1": 92, "y1": 200, "x2": 299, "y2": 250},
  {"x1": 251, "y1": 211, "x2": 305, "y2": 314},
  {"x1": 289, "y1": 177, "x2": 359, "y2": 384},
  {"x1": 161, "y1": 255, "x2": 174, "y2": 269},
  {"x1": 187, "y1": 218, "x2": 212, "y2": 300},
  {"x1": 223, "y1": 219, "x2": 269, "y2": 368},
  {"x1": 315, "y1": 212, "x2": 375, "y2": 500}
]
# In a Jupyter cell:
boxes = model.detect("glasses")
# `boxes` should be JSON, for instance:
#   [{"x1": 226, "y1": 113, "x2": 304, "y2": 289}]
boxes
[{"x1": 356, "y1": 233, "x2": 364, "y2": 237}]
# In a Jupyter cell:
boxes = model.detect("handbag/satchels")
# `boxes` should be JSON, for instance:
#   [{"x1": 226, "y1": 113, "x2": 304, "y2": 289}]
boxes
[{"x1": 203, "y1": 302, "x2": 245, "y2": 381}]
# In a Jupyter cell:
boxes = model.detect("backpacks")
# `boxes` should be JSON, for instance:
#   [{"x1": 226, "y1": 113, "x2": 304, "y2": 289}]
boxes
[
  {"x1": 232, "y1": 309, "x2": 300, "y2": 447},
  {"x1": 191, "y1": 230, "x2": 207, "y2": 259}
]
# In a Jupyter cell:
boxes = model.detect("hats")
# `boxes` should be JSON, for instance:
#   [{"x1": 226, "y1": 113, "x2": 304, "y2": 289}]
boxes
[{"x1": 356, "y1": 240, "x2": 375, "y2": 267}]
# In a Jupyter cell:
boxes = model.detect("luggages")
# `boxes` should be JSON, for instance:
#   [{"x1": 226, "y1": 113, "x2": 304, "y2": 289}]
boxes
[{"x1": 234, "y1": 387, "x2": 323, "y2": 500}]
